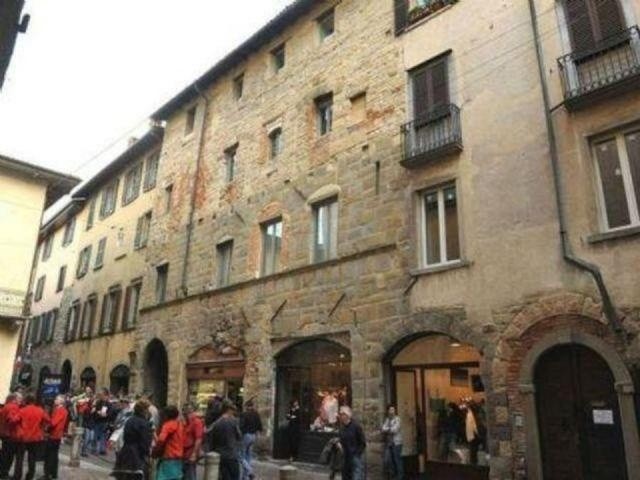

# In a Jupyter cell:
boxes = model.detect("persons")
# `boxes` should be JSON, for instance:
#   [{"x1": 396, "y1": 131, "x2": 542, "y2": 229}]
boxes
[
  {"x1": 378, "y1": 403, "x2": 405, "y2": 480},
  {"x1": 286, "y1": 399, "x2": 302, "y2": 462},
  {"x1": 0, "y1": 384, "x2": 263, "y2": 480},
  {"x1": 322, "y1": 405, "x2": 367, "y2": 480}
]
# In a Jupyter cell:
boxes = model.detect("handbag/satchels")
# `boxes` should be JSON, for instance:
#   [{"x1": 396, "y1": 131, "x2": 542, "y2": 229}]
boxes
[
  {"x1": 107, "y1": 428, "x2": 124, "y2": 454},
  {"x1": 151, "y1": 443, "x2": 165, "y2": 459}
]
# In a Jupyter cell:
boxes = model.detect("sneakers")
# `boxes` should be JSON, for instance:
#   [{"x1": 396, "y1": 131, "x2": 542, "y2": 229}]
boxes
[{"x1": 249, "y1": 472, "x2": 257, "y2": 480}]
